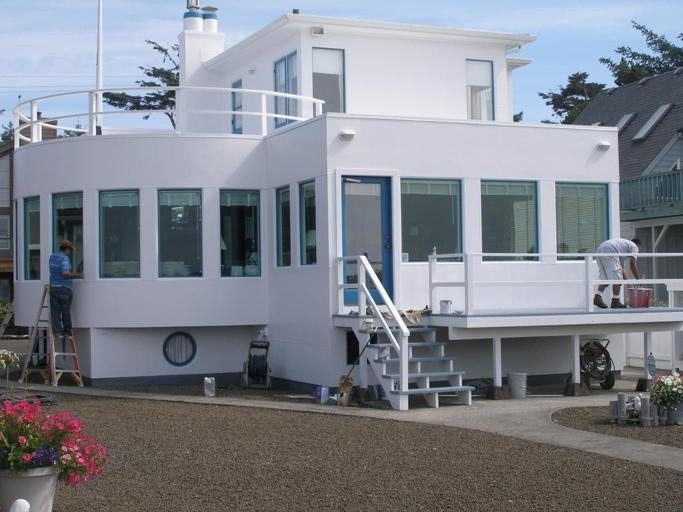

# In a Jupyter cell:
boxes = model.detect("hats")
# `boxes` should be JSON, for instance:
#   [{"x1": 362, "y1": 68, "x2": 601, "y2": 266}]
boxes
[{"x1": 62, "y1": 240, "x2": 74, "y2": 247}]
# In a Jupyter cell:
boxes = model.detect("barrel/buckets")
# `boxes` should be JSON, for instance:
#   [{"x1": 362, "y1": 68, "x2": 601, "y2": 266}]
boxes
[
  {"x1": 204, "y1": 376, "x2": 216, "y2": 397},
  {"x1": 506, "y1": 371, "x2": 528, "y2": 400},
  {"x1": 439, "y1": 299, "x2": 452, "y2": 314},
  {"x1": 627, "y1": 288, "x2": 653, "y2": 308}
]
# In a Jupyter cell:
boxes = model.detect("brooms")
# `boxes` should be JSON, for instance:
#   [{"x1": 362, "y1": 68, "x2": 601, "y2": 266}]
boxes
[{"x1": 327, "y1": 325, "x2": 379, "y2": 405}]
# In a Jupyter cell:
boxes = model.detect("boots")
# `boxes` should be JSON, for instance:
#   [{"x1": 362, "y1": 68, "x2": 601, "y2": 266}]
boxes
[
  {"x1": 611, "y1": 298, "x2": 626, "y2": 308},
  {"x1": 594, "y1": 294, "x2": 607, "y2": 308}
]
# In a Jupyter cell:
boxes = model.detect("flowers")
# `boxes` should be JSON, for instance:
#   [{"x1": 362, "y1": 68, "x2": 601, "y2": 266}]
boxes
[
  {"x1": 0, "y1": 396, "x2": 107, "y2": 488},
  {"x1": 648, "y1": 373, "x2": 682, "y2": 411}
]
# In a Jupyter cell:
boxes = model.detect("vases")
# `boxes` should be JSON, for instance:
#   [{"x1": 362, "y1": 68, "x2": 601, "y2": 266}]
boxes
[{"x1": 666, "y1": 400, "x2": 683, "y2": 425}]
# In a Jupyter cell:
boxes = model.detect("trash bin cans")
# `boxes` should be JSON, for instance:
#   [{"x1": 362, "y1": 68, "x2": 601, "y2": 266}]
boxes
[{"x1": 507, "y1": 372, "x2": 527, "y2": 399}]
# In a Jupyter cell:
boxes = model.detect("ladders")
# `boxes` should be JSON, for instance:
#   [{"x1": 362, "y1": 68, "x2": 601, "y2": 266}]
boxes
[{"x1": 18, "y1": 284, "x2": 84, "y2": 387}]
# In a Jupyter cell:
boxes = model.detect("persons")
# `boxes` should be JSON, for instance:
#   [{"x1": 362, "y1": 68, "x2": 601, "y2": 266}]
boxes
[
  {"x1": 49, "y1": 240, "x2": 80, "y2": 339},
  {"x1": 593, "y1": 238, "x2": 641, "y2": 308}
]
